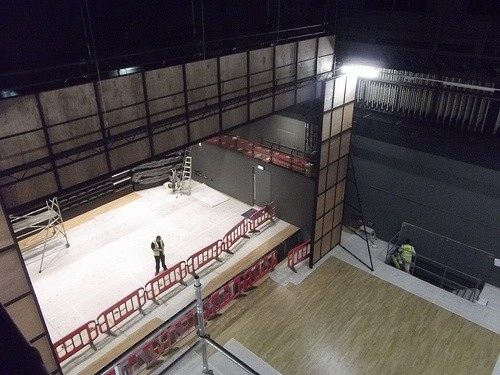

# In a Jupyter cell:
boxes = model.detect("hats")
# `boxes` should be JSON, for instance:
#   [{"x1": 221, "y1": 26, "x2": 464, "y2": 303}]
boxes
[{"x1": 156, "y1": 236, "x2": 161, "y2": 239}]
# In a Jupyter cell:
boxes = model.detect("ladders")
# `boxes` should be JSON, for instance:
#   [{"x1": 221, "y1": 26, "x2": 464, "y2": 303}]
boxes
[{"x1": 181, "y1": 157, "x2": 192, "y2": 194}]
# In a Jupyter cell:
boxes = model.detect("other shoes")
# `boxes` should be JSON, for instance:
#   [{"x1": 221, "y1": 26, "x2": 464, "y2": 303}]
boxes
[
  {"x1": 164, "y1": 268, "x2": 167, "y2": 270},
  {"x1": 155, "y1": 272, "x2": 159, "y2": 275}
]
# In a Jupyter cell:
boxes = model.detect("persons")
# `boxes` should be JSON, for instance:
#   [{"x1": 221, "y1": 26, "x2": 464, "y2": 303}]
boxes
[
  {"x1": 150, "y1": 236, "x2": 168, "y2": 276},
  {"x1": 396, "y1": 239, "x2": 417, "y2": 274},
  {"x1": 304, "y1": 158, "x2": 314, "y2": 179}
]
